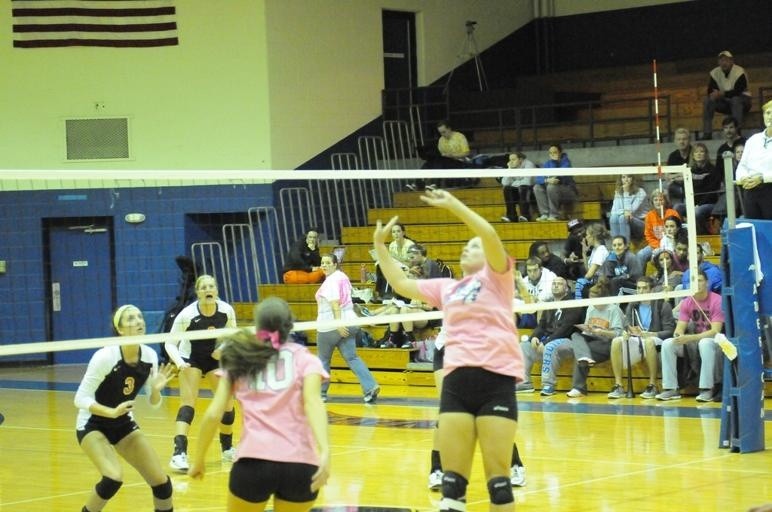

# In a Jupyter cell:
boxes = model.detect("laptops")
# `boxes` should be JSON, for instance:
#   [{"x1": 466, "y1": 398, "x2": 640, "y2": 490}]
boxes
[
  {"x1": 417, "y1": 143, "x2": 453, "y2": 162},
  {"x1": 332, "y1": 246, "x2": 346, "y2": 266}
]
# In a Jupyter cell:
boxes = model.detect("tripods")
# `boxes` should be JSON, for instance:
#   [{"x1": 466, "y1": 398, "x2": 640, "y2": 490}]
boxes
[{"x1": 442, "y1": 26, "x2": 489, "y2": 95}]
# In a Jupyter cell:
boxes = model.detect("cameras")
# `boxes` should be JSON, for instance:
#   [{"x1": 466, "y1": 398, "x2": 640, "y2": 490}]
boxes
[{"x1": 465, "y1": 20, "x2": 477, "y2": 26}]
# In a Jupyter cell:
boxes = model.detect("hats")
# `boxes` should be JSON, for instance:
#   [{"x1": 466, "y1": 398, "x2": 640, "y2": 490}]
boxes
[
  {"x1": 718, "y1": 50, "x2": 733, "y2": 58},
  {"x1": 567, "y1": 218, "x2": 585, "y2": 231}
]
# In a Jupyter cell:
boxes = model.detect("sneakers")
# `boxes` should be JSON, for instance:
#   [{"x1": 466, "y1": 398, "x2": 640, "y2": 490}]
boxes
[
  {"x1": 515, "y1": 384, "x2": 721, "y2": 402},
  {"x1": 168, "y1": 452, "x2": 189, "y2": 472},
  {"x1": 401, "y1": 336, "x2": 416, "y2": 349},
  {"x1": 428, "y1": 469, "x2": 445, "y2": 491},
  {"x1": 221, "y1": 447, "x2": 236, "y2": 462},
  {"x1": 510, "y1": 464, "x2": 527, "y2": 487},
  {"x1": 425, "y1": 184, "x2": 438, "y2": 191},
  {"x1": 363, "y1": 385, "x2": 380, "y2": 402},
  {"x1": 380, "y1": 336, "x2": 401, "y2": 349},
  {"x1": 404, "y1": 183, "x2": 417, "y2": 192},
  {"x1": 500, "y1": 215, "x2": 558, "y2": 222}
]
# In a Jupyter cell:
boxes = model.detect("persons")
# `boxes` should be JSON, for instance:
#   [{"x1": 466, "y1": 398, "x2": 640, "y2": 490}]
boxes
[
  {"x1": 74, "y1": 304, "x2": 179, "y2": 511},
  {"x1": 283, "y1": 230, "x2": 335, "y2": 284},
  {"x1": 373, "y1": 191, "x2": 528, "y2": 511},
  {"x1": 703, "y1": 51, "x2": 752, "y2": 142},
  {"x1": 222, "y1": 295, "x2": 333, "y2": 510},
  {"x1": 316, "y1": 251, "x2": 381, "y2": 403},
  {"x1": 165, "y1": 274, "x2": 238, "y2": 472},
  {"x1": 373, "y1": 100, "x2": 771, "y2": 401},
  {"x1": 406, "y1": 121, "x2": 468, "y2": 192}
]
